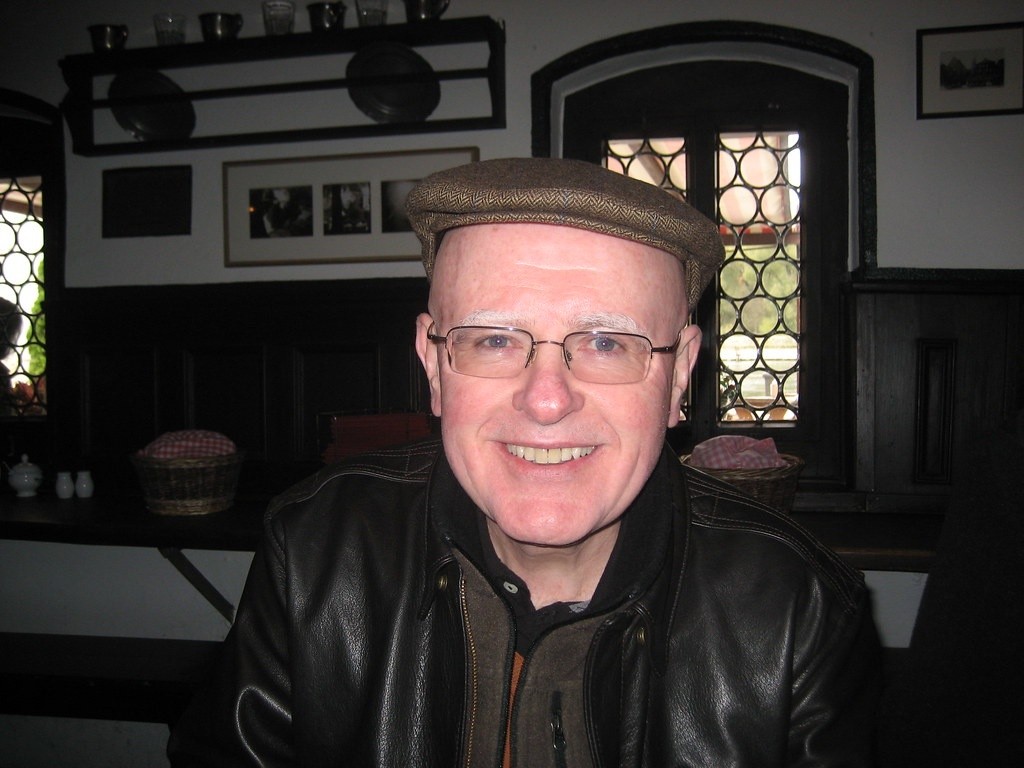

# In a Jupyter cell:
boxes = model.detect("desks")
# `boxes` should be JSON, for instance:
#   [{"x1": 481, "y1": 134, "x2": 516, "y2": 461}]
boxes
[{"x1": 0, "y1": 492, "x2": 950, "y2": 743}]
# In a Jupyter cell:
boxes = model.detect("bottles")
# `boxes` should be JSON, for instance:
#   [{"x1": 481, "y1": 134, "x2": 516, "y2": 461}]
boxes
[
  {"x1": 76, "y1": 472, "x2": 95, "y2": 497},
  {"x1": 56, "y1": 471, "x2": 73, "y2": 499},
  {"x1": 6, "y1": 454, "x2": 41, "y2": 496}
]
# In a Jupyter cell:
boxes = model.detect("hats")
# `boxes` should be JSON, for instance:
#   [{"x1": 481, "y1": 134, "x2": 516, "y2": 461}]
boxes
[{"x1": 406, "y1": 157, "x2": 726, "y2": 316}]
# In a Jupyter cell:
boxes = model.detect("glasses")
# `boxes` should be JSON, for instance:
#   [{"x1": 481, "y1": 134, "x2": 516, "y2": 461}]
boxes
[{"x1": 427, "y1": 321, "x2": 682, "y2": 385}]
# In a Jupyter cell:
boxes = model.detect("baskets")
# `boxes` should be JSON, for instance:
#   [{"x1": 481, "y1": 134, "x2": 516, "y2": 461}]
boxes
[
  {"x1": 680, "y1": 454, "x2": 804, "y2": 518},
  {"x1": 140, "y1": 449, "x2": 250, "y2": 515}
]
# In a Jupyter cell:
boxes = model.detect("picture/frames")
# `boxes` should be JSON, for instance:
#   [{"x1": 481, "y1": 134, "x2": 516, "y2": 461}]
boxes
[
  {"x1": 914, "y1": 21, "x2": 1024, "y2": 119},
  {"x1": 220, "y1": 147, "x2": 479, "y2": 267}
]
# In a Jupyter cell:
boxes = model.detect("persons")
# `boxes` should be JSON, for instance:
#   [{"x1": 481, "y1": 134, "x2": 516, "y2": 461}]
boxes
[
  {"x1": 0, "y1": 298, "x2": 24, "y2": 391},
  {"x1": 258, "y1": 183, "x2": 369, "y2": 238},
  {"x1": 168, "y1": 155, "x2": 892, "y2": 767}
]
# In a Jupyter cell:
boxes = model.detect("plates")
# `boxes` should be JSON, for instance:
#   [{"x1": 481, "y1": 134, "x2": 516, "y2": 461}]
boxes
[
  {"x1": 346, "y1": 40, "x2": 440, "y2": 122},
  {"x1": 105, "y1": 66, "x2": 196, "y2": 140}
]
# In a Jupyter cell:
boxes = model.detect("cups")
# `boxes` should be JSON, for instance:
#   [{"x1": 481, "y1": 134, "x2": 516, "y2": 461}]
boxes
[
  {"x1": 304, "y1": 2, "x2": 347, "y2": 32},
  {"x1": 355, "y1": 1, "x2": 389, "y2": 28},
  {"x1": 260, "y1": 1, "x2": 296, "y2": 36},
  {"x1": 199, "y1": 11, "x2": 242, "y2": 40},
  {"x1": 87, "y1": 24, "x2": 129, "y2": 52},
  {"x1": 153, "y1": 14, "x2": 187, "y2": 45},
  {"x1": 403, "y1": 0, "x2": 450, "y2": 24}
]
